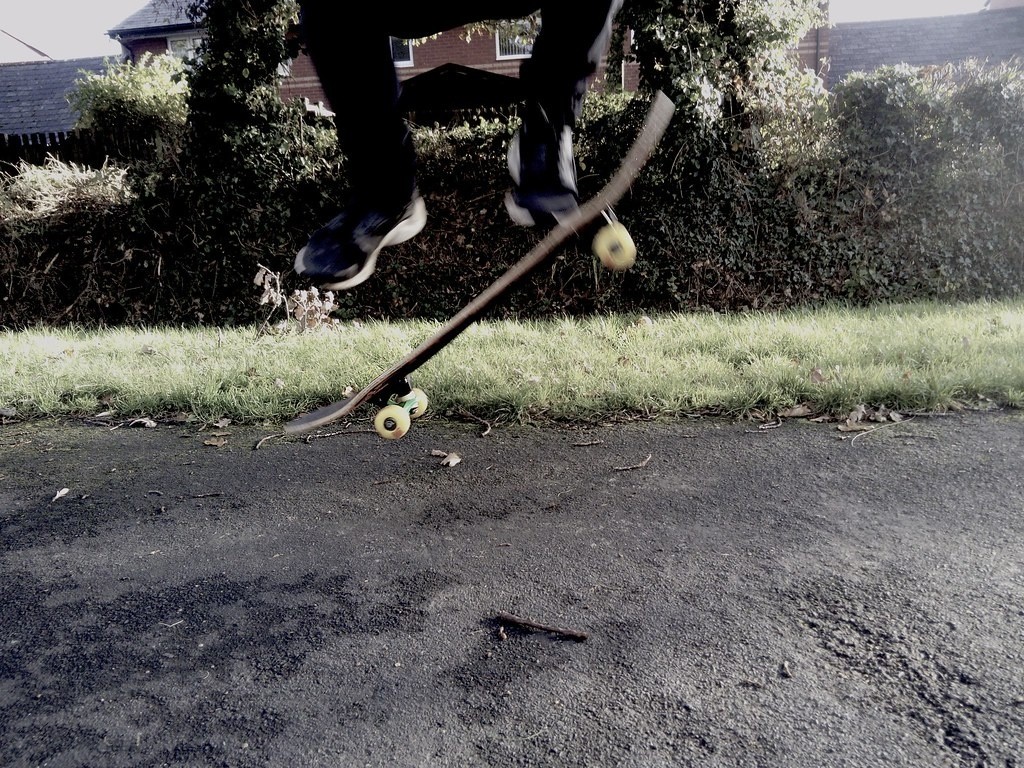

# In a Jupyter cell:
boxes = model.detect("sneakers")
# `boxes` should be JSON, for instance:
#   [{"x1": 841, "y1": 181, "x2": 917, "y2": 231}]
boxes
[
  {"x1": 503, "y1": 123, "x2": 581, "y2": 230},
  {"x1": 296, "y1": 196, "x2": 428, "y2": 290}
]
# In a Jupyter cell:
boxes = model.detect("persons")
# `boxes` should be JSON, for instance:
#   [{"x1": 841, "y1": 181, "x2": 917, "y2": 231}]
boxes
[{"x1": 295, "y1": 1, "x2": 625, "y2": 291}]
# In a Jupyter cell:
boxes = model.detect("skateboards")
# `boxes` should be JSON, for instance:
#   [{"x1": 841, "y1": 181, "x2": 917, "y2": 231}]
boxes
[{"x1": 282, "y1": 92, "x2": 675, "y2": 439}]
